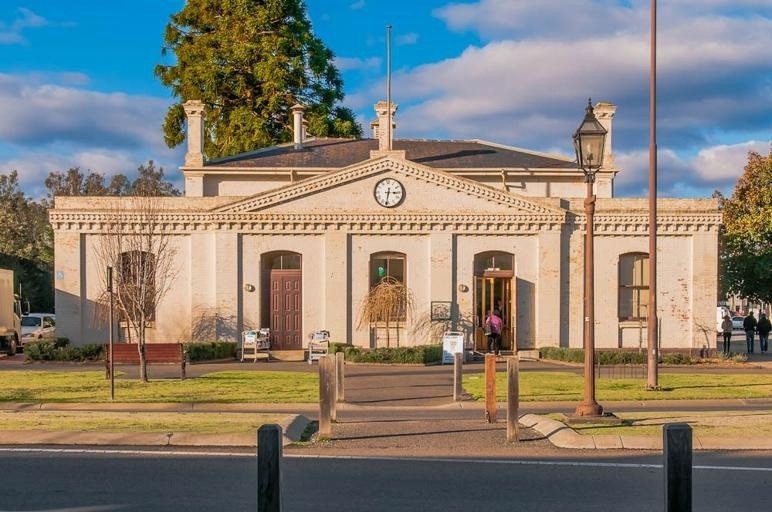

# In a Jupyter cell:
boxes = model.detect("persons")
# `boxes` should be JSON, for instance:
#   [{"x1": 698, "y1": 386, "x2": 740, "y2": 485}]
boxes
[
  {"x1": 743, "y1": 311, "x2": 772, "y2": 354},
  {"x1": 497, "y1": 299, "x2": 505, "y2": 316},
  {"x1": 485, "y1": 310, "x2": 502, "y2": 356},
  {"x1": 721, "y1": 315, "x2": 733, "y2": 353}
]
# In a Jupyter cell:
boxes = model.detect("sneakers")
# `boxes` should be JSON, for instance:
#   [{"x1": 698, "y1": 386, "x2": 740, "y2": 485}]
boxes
[{"x1": 722, "y1": 349, "x2": 768, "y2": 354}]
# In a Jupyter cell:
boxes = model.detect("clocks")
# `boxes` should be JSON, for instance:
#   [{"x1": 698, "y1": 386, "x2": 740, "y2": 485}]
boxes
[{"x1": 373, "y1": 178, "x2": 404, "y2": 208}]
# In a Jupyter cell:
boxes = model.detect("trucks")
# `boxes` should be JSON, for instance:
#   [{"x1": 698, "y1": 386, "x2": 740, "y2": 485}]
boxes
[
  {"x1": 717, "y1": 306, "x2": 734, "y2": 336},
  {"x1": 0, "y1": 267, "x2": 32, "y2": 357}
]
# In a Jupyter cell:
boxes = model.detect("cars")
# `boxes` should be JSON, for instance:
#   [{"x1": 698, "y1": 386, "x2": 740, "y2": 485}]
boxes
[
  {"x1": 732, "y1": 315, "x2": 747, "y2": 329},
  {"x1": 19, "y1": 310, "x2": 56, "y2": 353}
]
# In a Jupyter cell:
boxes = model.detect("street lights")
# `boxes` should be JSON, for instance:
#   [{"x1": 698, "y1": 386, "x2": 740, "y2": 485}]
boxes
[{"x1": 568, "y1": 93, "x2": 613, "y2": 420}]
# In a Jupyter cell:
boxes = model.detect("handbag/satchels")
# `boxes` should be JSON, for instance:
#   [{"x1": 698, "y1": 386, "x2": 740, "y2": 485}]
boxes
[{"x1": 485, "y1": 322, "x2": 492, "y2": 336}]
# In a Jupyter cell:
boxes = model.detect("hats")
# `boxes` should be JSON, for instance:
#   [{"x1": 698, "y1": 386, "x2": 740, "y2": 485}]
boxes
[{"x1": 722, "y1": 315, "x2": 730, "y2": 319}]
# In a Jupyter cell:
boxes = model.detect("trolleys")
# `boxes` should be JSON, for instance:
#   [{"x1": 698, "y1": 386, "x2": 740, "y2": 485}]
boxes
[
  {"x1": 239, "y1": 327, "x2": 274, "y2": 364},
  {"x1": 306, "y1": 331, "x2": 329, "y2": 365}
]
